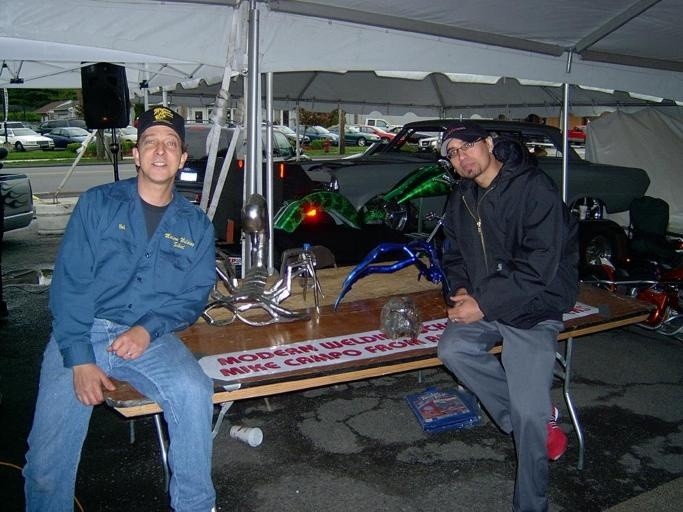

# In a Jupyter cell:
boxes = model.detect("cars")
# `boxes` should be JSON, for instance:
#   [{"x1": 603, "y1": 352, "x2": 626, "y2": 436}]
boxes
[{"x1": 142, "y1": 122, "x2": 316, "y2": 251}]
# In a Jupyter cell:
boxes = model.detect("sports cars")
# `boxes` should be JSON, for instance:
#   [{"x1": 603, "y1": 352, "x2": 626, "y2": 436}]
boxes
[{"x1": 233, "y1": 118, "x2": 652, "y2": 236}]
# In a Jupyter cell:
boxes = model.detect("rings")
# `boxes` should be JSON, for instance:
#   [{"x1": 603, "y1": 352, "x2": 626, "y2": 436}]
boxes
[
  {"x1": 127, "y1": 351, "x2": 132, "y2": 356},
  {"x1": 454, "y1": 318, "x2": 458, "y2": 323}
]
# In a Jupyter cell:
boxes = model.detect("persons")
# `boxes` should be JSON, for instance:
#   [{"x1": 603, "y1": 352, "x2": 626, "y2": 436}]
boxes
[
  {"x1": 433, "y1": 123, "x2": 582, "y2": 510},
  {"x1": 22, "y1": 102, "x2": 226, "y2": 512}
]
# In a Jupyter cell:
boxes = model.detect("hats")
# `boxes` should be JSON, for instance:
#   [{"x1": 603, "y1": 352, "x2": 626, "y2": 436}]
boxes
[
  {"x1": 136, "y1": 106, "x2": 187, "y2": 143},
  {"x1": 440, "y1": 118, "x2": 488, "y2": 157}
]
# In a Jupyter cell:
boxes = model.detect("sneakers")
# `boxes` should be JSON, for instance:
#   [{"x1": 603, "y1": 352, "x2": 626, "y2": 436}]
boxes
[{"x1": 546, "y1": 405, "x2": 568, "y2": 462}]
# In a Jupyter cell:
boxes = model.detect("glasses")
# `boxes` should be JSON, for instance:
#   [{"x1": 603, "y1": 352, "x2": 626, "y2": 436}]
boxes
[{"x1": 448, "y1": 139, "x2": 482, "y2": 157}]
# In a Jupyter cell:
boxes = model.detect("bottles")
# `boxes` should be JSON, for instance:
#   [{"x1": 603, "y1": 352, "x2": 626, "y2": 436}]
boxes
[{"x1": 298, "y1": 243, "x2": 316, "y2": 287}]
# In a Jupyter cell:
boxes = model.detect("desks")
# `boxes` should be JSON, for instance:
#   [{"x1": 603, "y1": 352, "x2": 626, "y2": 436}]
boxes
[{"x1": 103, "y1": 253, "x2": 658, "y2": 512}]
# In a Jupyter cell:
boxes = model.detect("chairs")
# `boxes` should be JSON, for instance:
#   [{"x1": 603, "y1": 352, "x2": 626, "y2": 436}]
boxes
[{"x1": 280, "y1": 244, "x2": 338, "y2": 275}]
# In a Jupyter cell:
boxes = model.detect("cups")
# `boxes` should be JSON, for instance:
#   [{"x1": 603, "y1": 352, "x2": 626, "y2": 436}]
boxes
[{"x1": 229, "y1": 424, "x2": 263, "y2": 448}]
